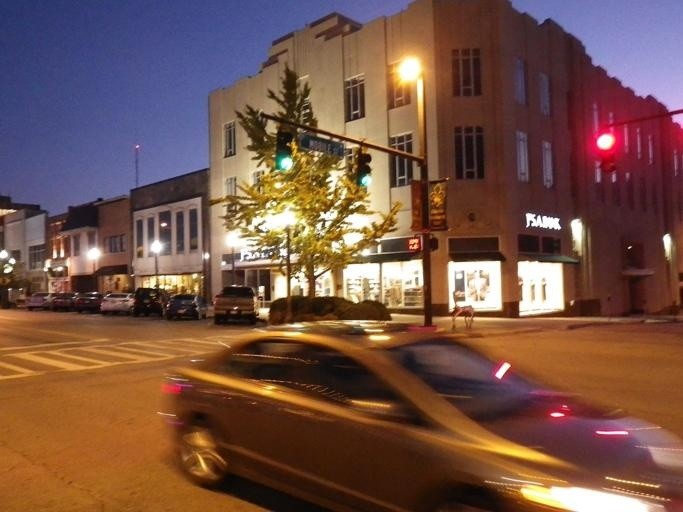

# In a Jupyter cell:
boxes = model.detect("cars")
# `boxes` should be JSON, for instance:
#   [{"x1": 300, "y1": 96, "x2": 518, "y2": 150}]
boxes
[
  {"x1": 28, "y1": 286, "x2": 208, "y2": 322},
  {"x1": 156, "y1": 321, "x2": 682, "y2": 509}
]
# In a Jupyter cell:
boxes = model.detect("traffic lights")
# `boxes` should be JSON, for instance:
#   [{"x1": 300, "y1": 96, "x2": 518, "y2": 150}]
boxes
[
  {"x1": 277, "y1": 130, "x2": 293, "y2": 171},
  {"x1": 356, "y1": 153, "x2": 372, "y2": 186},
  {"x1": 595, "y1": 130, "x2": 618, "y2": 154},
  {"x1": 407, "y1": 236, "x2": 422, "y2": 252}
]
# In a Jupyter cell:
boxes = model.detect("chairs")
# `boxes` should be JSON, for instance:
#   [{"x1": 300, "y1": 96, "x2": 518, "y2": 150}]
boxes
[{"x1": 451, "y1": 292, "x2": 475, "y2": 330}]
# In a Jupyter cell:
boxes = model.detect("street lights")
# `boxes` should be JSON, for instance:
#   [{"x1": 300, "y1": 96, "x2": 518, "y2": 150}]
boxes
[
  {"x1": 0, "y1": 249, "x2": 16, "y2": 287},
  {"x1": 150, "y1": 240, "x2": 162, "y2": 288},
  {"x1": 397, "y1": 56, "x2": 432, "y2": 327},
  {"x1": 87, "y1": 248, "x2": 101, "y2": 275}
]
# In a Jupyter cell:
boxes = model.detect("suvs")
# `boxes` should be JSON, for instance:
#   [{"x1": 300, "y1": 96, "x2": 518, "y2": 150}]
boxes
[{"x1": 212, "y1": 285, "x2": 263, "y2": 327}]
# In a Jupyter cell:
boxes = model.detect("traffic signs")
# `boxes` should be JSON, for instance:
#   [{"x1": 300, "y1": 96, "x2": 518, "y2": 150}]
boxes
[{"x1": 300, "y1": 133, "x2": 344, "y2": 160}]
